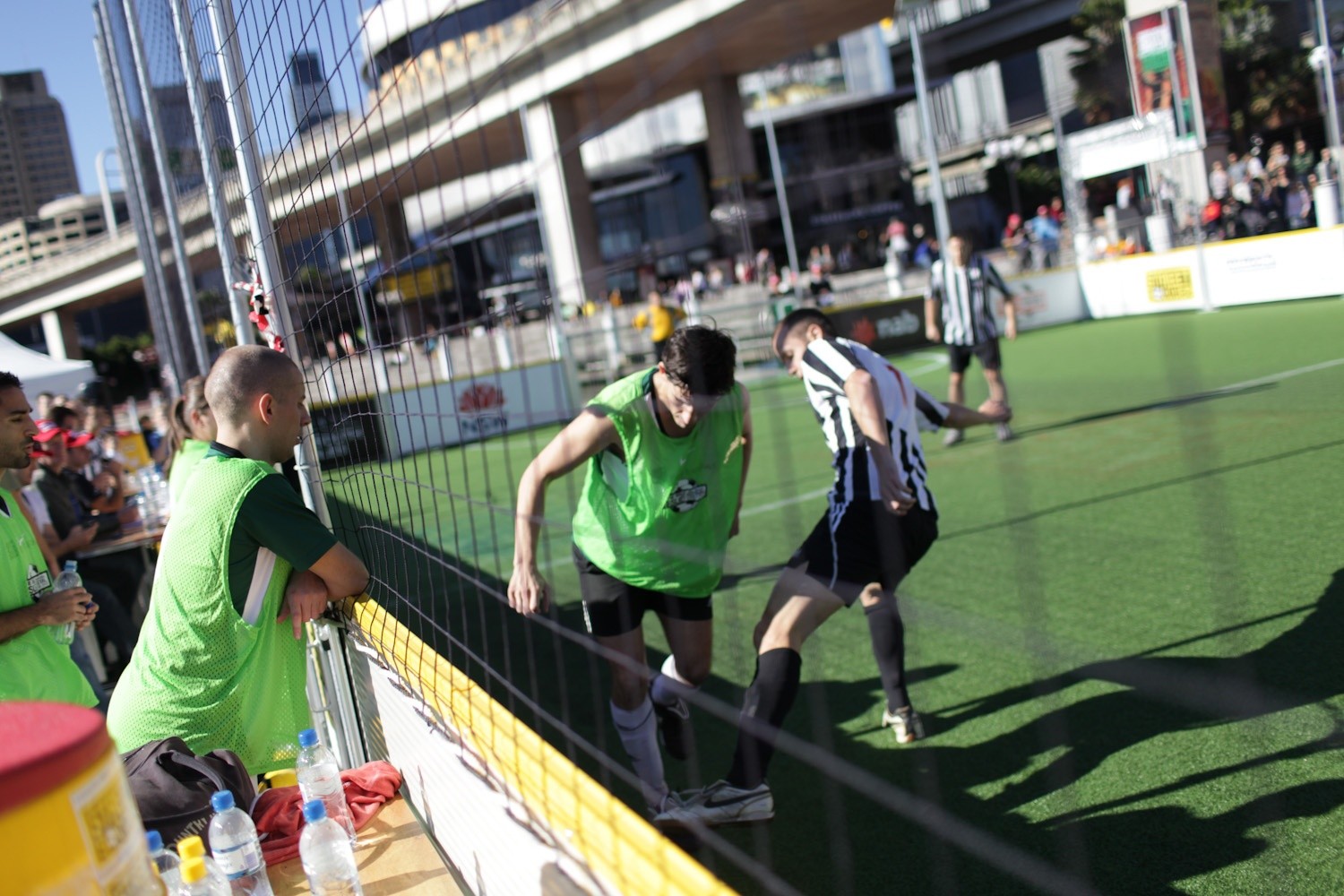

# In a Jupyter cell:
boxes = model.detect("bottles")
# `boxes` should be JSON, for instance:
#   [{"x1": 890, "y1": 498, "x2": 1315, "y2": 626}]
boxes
[
  {"x1": 48, "y1": 560, "x2": 83, "y2": 644},
  {"x1": 299, "y1": 800, "x2": 364, "y2": 896},
  {"x1": 208, "y1": 790, "x2": 273, "y2": 896},
  {"x1": 179, "y1": 858, "x2": 222, "y2": 896},
  {"x1": 297, "y1": 728, "x2": 356, "y2": 850},
  {"x1": 149, "y1": 831, "x2": 180, "y2": 895},
  {"x1": 177, "y1": 836, "x2": 232, "y2": 896},
  {"x1": 123, "y1": 463, "x2": 170, "y2": 526}
]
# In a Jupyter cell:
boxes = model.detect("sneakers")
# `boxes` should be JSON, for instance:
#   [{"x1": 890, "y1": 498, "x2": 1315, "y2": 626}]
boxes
[
  {"x1": 648, "y1": 791, "x2": 690, "y2": 836},
  {"x1": 654, "y1": 776, "x2": 777, "y2": 829},
  {"x1": 943, "y1": 428, "x2": 964, "y2": 448},
  {"x1": 995, "y1": 421, "x2": 1011, "y2": 441},
  {"x1": 646, "y1": 675, "x2": 697, "y2": 759},
  {"x1": 881, "y1": 708, "x2": 926, "y2": 746}
]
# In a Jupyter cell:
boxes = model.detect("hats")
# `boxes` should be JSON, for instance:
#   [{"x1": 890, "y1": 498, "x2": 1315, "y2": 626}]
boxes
[{"x1": 21, "y1": 416, "x2": 95, "y2": 459}]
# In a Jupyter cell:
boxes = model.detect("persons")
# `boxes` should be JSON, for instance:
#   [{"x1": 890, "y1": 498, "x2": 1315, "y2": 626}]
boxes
[
  {"x1": 683, "y1": 309, "x2": 1014, "y2": 823},
  {"x1": 0, "y1": 345, "x2": 372, "y2": 778},
  {"x1": 507, "y1": 326, "x2": 751, "y2": 813},
  {"x1": 641, "y1": 196, "x2": 1069, "y2": 364},
  {"x1": 1208, "y1": 140, "x2": 1337, "y2": 238},
  {"x1": 924, "y1": 232, "x2": 1032, "y2": 445}
]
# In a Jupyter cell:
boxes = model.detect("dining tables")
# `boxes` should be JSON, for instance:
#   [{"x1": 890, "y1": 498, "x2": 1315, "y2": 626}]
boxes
[
  {"x1": 261, "y1": 789, "x2": 465, "y2": 895},
  {"x1": 75, "y1": 464, "x2": 168, "y2": 572}
]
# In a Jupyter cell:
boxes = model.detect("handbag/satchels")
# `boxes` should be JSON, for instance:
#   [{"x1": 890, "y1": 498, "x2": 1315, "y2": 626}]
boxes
[{"x1": 121, "y1": 736, "x2": 259, "y2": 869}]
